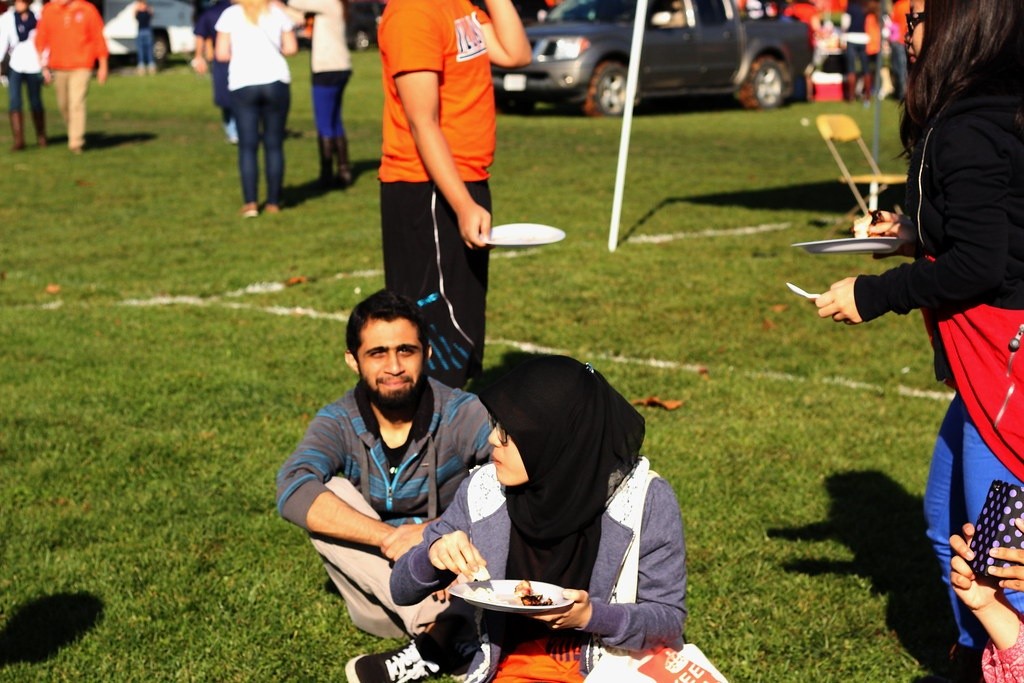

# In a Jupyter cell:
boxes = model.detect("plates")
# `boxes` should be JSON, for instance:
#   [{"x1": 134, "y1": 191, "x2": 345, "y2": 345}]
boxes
[
  {"x1": 447, "y1": 580, "x2": 575, "y2": 614},
  {"x1": 478, "y1": 223, "x2": 567, "y2": 246},
  {"x1": 791, "y1": 237, "x2": 907, "y2": 254}
]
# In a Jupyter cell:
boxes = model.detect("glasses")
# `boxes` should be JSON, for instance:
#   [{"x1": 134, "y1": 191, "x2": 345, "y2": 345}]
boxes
[
  {"x1": 488, "y1": 412, "x2": 507, "y2": 443},
  {"x1": 906, "y1": 12, "x2": 925, "y2": 35}
]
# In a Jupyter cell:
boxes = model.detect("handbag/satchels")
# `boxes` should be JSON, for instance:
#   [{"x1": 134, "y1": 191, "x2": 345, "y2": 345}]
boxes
[{"x1": 583, "y1": 470, "x2": 729, "y2": 683}]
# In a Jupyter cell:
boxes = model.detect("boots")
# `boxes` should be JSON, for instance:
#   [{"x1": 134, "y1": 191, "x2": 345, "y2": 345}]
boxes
[
  {"x1": 311, "y1": 137, "x2": 334, "y2": 188},
  {"x1": 846, "y1": 72, "x2": 856, "y2": 101},
  {"x1": 863, "y1": 74, "x2": 873, "y2": 100},
  {"x1": 10, "y1": 111, "x2": 26, "y2": 150},
  {"x1": 33, "y1": 111, "x2": 47, "y2": 146},
  {"x1": 335, "y1": 135, "x2": 352, "y2": 186}
]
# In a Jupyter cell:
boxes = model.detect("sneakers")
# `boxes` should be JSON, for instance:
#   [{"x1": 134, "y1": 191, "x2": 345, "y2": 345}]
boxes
[
  {"x1": 345, "y1": 640, "x2": 440, "y2": 683},
  {"x1": 445, "y1": 630, "x2": 481, "y2": 675}
]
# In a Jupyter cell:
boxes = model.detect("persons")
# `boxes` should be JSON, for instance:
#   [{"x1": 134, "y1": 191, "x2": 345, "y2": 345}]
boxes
[
  {"x1": 134, "y1": 0, "x2": 154, "y2": 66},
  {"x1": 275, "y1": 290, "x2": 494, "y2": 683},
  {"x1": 191, "y1": 0, "x2": 354, "y2": 218},
  {"x1": 0, "y1": 0, "x2": 109, "y2": 153},
  {"x1": 390, "y1": 352, "x2": 686, "y2": 683},
  {"x1": 807, "y1": 0, "x2": 1024, "y2": 683},
  {"x1": 377, "y1": 0, "x2": 532, "y2": 384},
  {"x1": 948, "y1": 517, "x2": 1024, "y2": 683},
  {"x1": 736, "y1": 0, "x2": 909, "y2": 100}
]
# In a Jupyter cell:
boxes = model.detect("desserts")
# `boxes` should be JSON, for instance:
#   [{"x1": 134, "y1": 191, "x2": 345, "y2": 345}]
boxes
[
  {"x1": 853, "y1": 211, "x2": 913, "y2": 239},
  {"x1": 458, "y1": 566, "x2": 554, "y2": 605}
]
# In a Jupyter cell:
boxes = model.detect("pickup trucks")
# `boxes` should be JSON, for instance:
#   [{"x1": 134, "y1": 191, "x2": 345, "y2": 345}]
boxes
[{"x1": 491, "y1": 0, "x2": 813, "y2": 117}]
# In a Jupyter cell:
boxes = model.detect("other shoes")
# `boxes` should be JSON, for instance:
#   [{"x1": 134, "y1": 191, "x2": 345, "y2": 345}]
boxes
[
  {"x1": 69, "y1": 146, "x2": 81, "y2": 154},
  {"x1": 224, "y1": 118, "x2": 240, "y2": 144},
  {"x1": 136, "y1": 68, "x2": 146, "y2": 76},
  {"x1": 927, "y1": 634, "x2": 977, "y2": 683},
  {"x1": 265, "y1": 203, "x2": 279, "y2": 213},
  {"x1": 240, "y1": 202, "x2": 259, "y2": 217},
  {"x1": 148, "y1": 67, "x2": 155, "y2": 76}
]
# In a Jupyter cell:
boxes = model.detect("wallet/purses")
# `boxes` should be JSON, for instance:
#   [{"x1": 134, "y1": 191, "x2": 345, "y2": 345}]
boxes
[{"x1": 967, "y1": 480, "x2": 1024, "y2": 579}]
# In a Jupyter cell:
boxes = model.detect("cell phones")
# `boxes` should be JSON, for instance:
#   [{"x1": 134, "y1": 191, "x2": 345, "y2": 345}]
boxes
[{"x1": 966, "y1": 479, "x2": 1024, "y2": 576}]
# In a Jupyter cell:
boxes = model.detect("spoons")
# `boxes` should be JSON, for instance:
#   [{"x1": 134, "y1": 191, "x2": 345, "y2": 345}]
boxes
[{"x1": 785, "y1": 282, "x2": 821, "y2": 299}]
